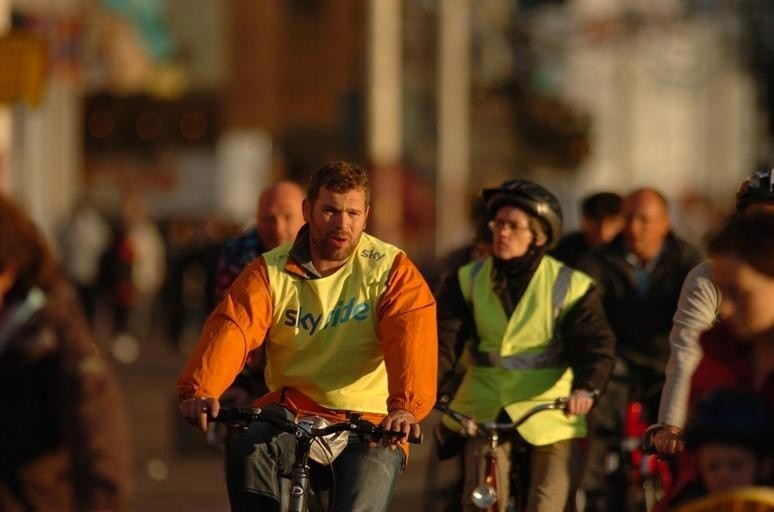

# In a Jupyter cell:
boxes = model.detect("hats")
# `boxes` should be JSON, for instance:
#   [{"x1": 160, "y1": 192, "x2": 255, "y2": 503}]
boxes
[
  {"x1": 708, "y1": 214, "x2": 773, "y2": 277},
  {"x1": 581, "y1": 192, "x2": 623, "y2": 214}
]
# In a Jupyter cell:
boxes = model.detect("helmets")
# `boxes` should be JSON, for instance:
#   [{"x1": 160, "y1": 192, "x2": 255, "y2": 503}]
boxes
[
  {"x1": 482, "y1": 179, "x2": 564, "y2": 251},
  {"x1": 735, "y1": 170, "x2": 774, "y2": 201}
]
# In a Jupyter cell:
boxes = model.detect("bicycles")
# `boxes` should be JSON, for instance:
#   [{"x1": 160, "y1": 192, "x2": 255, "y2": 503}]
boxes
[
  {"x1": 431, "y1": 387, "x2": 601, "y2": 511},
  {"x1": 173, "y1": 401, "x2": 425, "y2": 511},
  {"x1": 639, "y1": 423, "x2": 774, "y2": 512}
]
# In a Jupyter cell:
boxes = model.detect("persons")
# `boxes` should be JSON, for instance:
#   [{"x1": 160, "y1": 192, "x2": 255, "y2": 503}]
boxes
[
  {"x1": 691, "y1": 210, "x2": 774, "y2": 423},
  {"x1": 56, "y1": 212, "x2": 243, "y2": 345},
  {"x1": 537, "y1": 191, "x2": 627, "y2": 269},
  {"x1": 211, "y1": 179, "x2": 305, "y2": 447},
  {"x1": 178, "y1": 161, "x2": 438, "y2": 512},
  {"x1": 0, "y1": 195, "x2": 134, "y2": 512},
  {"x1": 659, "y1": 389, "x2": 773, "y2": 511},
  {"x1": 432, "y1": 179, "x2": 618, "y2": 512},
  {"x1": 590, "y1": 187, "x2": 704, "y2": 486},
  {"x1": 434, "y1": 189, "x2": 499, "y2": 290},
  {"x1": 644, "y1": 170, "x2": 774, "y2": 462}
]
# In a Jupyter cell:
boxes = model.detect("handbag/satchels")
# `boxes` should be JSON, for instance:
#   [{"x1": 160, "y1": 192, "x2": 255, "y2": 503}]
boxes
[{"x1": 296, "y1": 416, "x2": 349, "y2": 466}]
